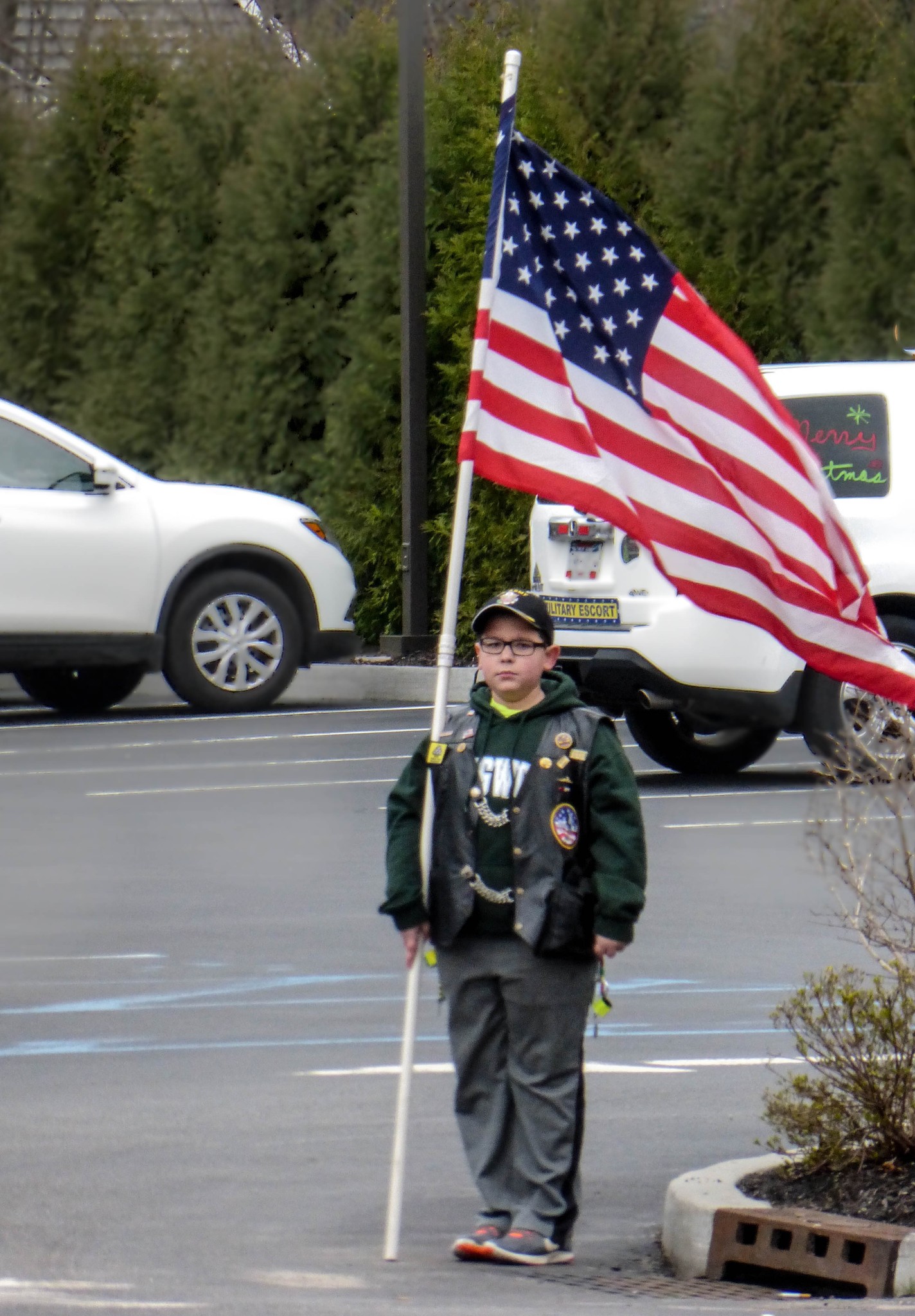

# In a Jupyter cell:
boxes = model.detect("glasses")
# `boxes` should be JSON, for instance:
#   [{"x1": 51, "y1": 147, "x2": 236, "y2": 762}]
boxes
[{"x1": 477, "y1": 636, "x2": 545, "y2": 656}]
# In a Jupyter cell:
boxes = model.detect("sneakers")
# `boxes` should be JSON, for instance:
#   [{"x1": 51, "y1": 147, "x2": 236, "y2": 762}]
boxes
[
  {"x1": 452, "y1": 1226, "x2": 501, "y2": 1262},
  {"x1": 480, "y1": 1229, "x2": 575, "y2": 1266}
]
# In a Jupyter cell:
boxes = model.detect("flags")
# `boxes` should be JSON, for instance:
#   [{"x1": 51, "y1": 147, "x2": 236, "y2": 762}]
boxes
[{"x1": 456, "y1": 127, "x2": 915, "y2": 713}]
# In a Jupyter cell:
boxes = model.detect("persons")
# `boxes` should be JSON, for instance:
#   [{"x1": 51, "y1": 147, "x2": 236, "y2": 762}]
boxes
[{"x1": 377, "y1": 589, "x2": 647, "y2": 1266}]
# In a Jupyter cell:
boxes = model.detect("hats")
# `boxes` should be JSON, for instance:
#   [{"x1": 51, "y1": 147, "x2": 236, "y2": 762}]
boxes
[{"x1": 471, "y1": 587, "x2": 555, "y2": 647}]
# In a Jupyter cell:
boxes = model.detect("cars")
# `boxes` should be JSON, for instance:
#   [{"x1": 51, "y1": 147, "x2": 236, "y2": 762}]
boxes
[
  {"x1": 0, "y1": 396, "x2": 367, "y2": 711},
  {"x1": 530, "y1": 361, "x2": 915, "y2": 786}
]
